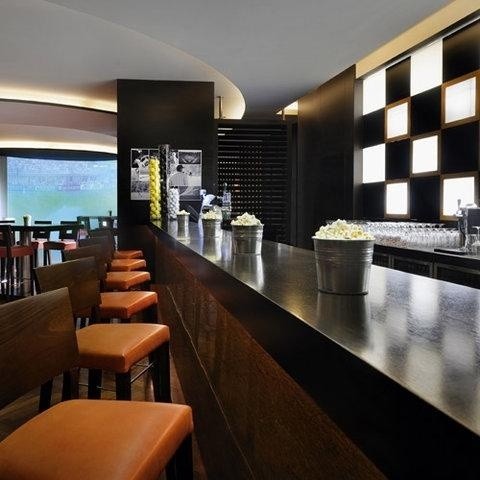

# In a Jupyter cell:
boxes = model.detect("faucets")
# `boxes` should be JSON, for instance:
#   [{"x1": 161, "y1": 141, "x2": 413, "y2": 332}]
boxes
[{"x1": 454, "y1": 207, "x2": 469, "y2": 248}]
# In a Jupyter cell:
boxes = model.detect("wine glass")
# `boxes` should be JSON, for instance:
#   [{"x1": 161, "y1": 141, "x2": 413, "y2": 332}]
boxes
[{"x1": 354, "y1": 222, "x2": 462, "y2": 251}]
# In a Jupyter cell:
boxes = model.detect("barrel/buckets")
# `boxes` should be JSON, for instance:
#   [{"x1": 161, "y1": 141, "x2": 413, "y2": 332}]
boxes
[
  {"x1": 177, "y1": 215, "x2": 189, "y2": 227},
  {"x1": 231, "y1": 224, "x2": 264, "y2": 256},
  {"x1": 203, "y1": 237, "x2": 221, "y2": 258},
  {"x1": 311, "y1": 236, "x2": 376, "y2": 296},
  {"x1": 317, "y1": 289, "x2": 372, "y2": 352},
  {"x1": 233, "y1": 256, "x2": 264, "y2": 291},
  {"x1": 202, "y1": 219, "x2": 222, "y2": 237},
  {"x1": 177, "y1": 228, "x2": 190, "y2": 238}
]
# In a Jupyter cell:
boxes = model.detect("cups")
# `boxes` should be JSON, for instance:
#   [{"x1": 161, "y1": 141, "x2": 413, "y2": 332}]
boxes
[
  {"x1": 24, "y1": 216, "x2": 31, "y2": 226},
  {"x1": 465, "y1": 234, "x2": 477, "y2": 255}
]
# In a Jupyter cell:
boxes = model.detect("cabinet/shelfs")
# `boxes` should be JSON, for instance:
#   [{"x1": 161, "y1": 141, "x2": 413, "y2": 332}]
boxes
[{"x1": 373, "y1": 252, "x2": 480, "y2": 288}]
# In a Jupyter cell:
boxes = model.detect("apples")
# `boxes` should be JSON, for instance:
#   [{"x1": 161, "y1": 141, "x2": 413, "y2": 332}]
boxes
[{"x1": 149, "y1": 159, "x2": 161, "y2": 219}]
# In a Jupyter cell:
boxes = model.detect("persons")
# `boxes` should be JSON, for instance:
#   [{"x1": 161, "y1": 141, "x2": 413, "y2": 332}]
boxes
[{"x1": 168, "y1": 164, "x2": 189, "y2": 186}]
